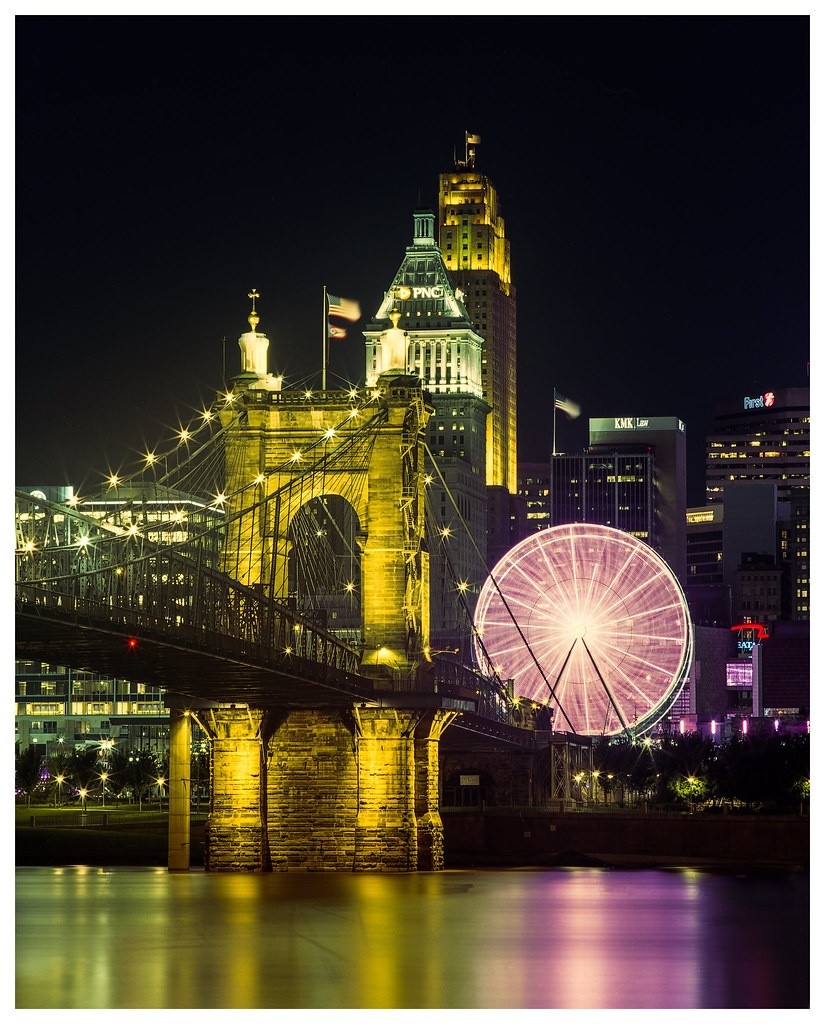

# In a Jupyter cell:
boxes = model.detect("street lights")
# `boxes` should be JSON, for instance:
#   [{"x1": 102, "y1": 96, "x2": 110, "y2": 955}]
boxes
[
  {"x1": 79, "y1": 789, "x2": 87, "y2": 813},
  {"x1": 157, "y1": 778, "x2": 164, "y2": 812},
  {"x1": 593, "y1": 772, "x2": 599, "y2": 804},
  {"x1": 57, "y1": 777, "x2": 63, "y2": 808},
  {"x1": 607, "y1": 774, "x2": 614, "y2": 806},
  {"x1": 575, "y1": 776, "x2": 581, "y2": 802},
  {"x1": 99, "y1": 772, "x2": 109, "y2": 807}
]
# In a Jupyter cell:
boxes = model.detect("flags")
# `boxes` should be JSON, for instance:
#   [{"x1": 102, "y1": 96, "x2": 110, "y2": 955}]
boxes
[
  {"x1": 326, "y1": 294, "x2": 361, "y2": 322},
  {"x1": 466, "y1": 133, "x2": 482, "y2": 144},
  {"x1": 327, "y1": 324, "x2": 347, "y2": 338},
  {"x1": 552, "y1": 391, "x2": 580, "y2": 417}
]
